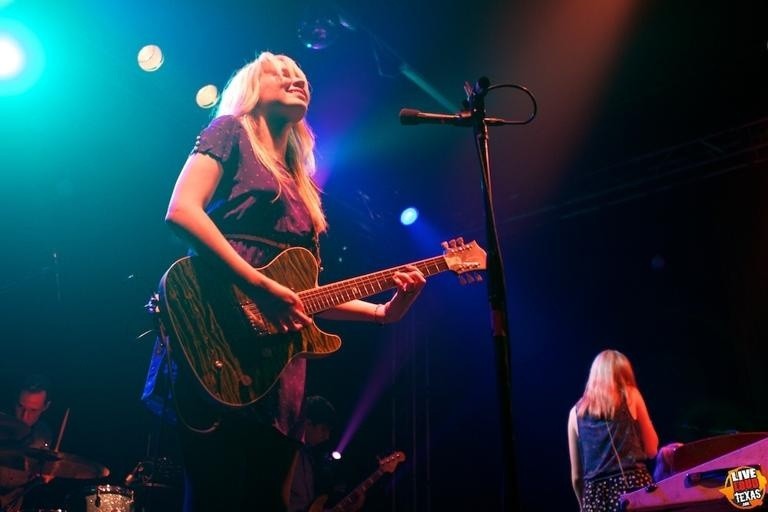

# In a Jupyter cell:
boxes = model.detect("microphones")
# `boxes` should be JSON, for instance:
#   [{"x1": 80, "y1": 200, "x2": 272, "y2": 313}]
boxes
[{"x1": 398, "y1": 109, "x2": 503, "y2": 127}]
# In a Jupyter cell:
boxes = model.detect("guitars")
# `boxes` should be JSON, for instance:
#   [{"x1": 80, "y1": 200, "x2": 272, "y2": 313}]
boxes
[
  {"x1": 308, "y1": 452, "x2": 406, "y2": 512},
  {"x1": 157, "y1": 236, "x2": 487, "y2": 406}
]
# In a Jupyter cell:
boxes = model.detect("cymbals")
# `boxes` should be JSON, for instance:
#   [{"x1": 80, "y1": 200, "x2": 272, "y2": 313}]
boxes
[{"x1": 1, "y1": 446, "x2": 110, "y2": 478}]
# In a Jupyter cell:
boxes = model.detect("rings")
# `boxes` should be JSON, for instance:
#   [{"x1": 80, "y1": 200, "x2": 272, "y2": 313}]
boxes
[{"x1": 405, "y1": 291, "x2": 415, "y2": 298}]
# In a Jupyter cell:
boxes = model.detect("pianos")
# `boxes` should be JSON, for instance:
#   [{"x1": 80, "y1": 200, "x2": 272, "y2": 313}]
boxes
[{"x1": 619, "y1": 432, "x2": 768, "y2": 510}]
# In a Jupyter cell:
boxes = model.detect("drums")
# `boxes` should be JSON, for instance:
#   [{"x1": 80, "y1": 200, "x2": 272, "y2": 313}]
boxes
[{"x1": 85, "y1": 485, "x2": 134, "y2": 512}]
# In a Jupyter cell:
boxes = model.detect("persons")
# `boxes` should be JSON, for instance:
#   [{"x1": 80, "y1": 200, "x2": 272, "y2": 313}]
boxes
[
  {"x1": 566, "y1": 348, "x2": 660, "y2": 512},
  {"x1": 140, "y1": 49, "x2": 428, "y2": 511},
  {"x1": 1, "y1": 380, "x2": 54, "y2": 452},
  {"x1": 285, "y1": 395, "x2": 366, "y2": 512}
]
